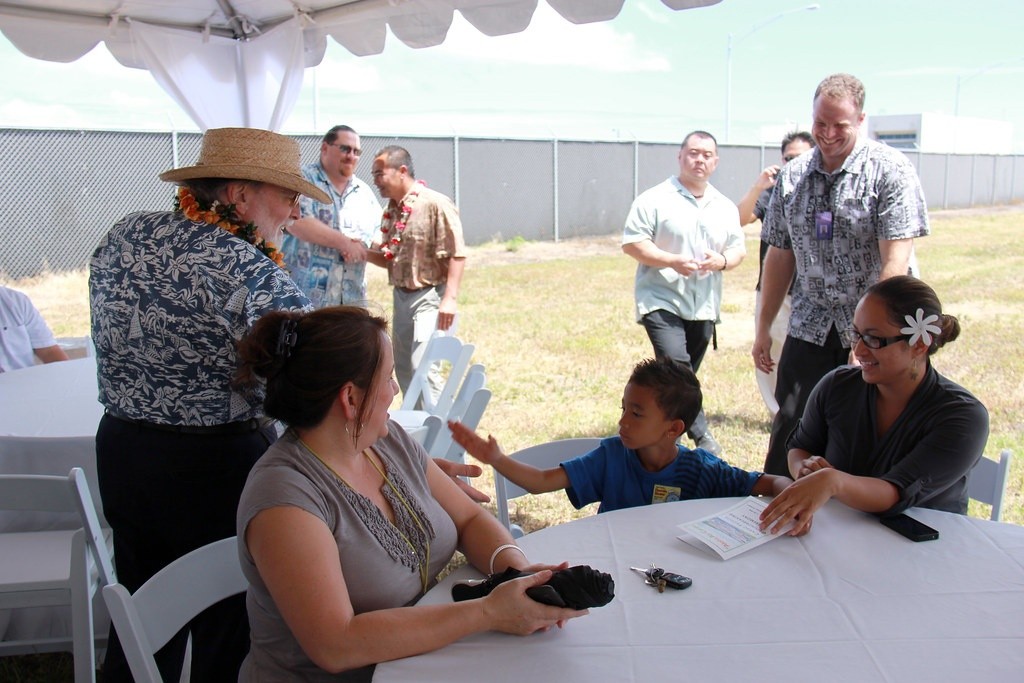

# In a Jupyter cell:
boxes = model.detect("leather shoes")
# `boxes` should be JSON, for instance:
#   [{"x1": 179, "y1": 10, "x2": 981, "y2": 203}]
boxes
[{"x1": 696, "y1": 430, "x2": 722, "y2": 457}]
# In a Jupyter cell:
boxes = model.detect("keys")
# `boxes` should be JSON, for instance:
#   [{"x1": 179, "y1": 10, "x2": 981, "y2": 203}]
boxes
[{"x1": 629, "y1": 565, "x2": 692, "y2": 592}]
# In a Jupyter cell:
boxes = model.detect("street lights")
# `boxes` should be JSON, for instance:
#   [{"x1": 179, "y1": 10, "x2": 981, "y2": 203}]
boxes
[{"x1": 723, "y1": 6, "x2": 818, "y2": 144}]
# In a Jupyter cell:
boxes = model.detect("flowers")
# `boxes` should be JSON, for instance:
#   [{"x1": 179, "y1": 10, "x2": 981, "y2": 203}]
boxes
[{"x1": 901, "y1": 308, "x2": 942, "y2": 347}]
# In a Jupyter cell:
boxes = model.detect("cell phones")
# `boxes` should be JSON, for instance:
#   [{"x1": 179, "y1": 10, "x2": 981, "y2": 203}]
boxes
[
  {"x1": 526, "y1": 585, "x2": 566, "y2": 608},
  {"x1": 880, "y1": 513, "x2": 939, "y2": 543}
]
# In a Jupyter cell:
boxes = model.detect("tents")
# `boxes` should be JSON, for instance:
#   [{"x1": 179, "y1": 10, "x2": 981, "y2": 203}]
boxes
[{"x1": 0, "y1": 0, "x2": 721, "y2": 133}]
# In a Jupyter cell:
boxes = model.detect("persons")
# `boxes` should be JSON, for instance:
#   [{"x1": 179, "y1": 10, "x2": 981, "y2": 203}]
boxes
[
  {"x1": 237, "y1": 307, "x2": 589, "y2": 683},
  {"x1": 88, "y1": 127, "x2": 334, "y2": 683},
  {"x1": 760, "y1": 276, "x2": 989, "y2": 536},
  {"x1": 623, "y1": 131, "x2": 747, "y2": 456},
  {"x1": 0, "y1": 286, "x2": 69, "y2": 375},
  {"x1": 447, "y1": 359, "x2": 812, "y2": 538},
  {"x1": 342, "y1": 144, "x2": 471, "y2": 486},
  {"x1": 738, "y1": 131, "x2": 818, "y2": 418},
  {"x1": 751, "y1": 74, "x2": 931, "y2": 481},
  {"x1": 276, "y1": 125, "x2": 383, "y2": 314}
]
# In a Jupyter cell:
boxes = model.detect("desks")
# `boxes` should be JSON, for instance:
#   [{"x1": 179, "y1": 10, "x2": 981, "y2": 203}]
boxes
[
  {"x1": 374, "y1": 496, "x2": 1024, "y2": 683},
  {"x1": 0, "y1": 357, "x2": 290, "y2": 682}
]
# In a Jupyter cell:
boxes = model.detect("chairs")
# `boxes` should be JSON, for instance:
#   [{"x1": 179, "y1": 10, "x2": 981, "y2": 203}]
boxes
[
  {"x1": 54, "y1": 336, "x2": 97, "y2": 357},
  {"x1": 493, "y1": 438, "x2": 606, "y2": 539},
  {"x1": 384, "y1": 330, "x2": 492, "y2": 486},
  {"x1": 966, "y1": 450, "x2": 1010, "y2": 521},
  {"x1": 0, "y1": 468, "x2": 250, "y2": 683}
]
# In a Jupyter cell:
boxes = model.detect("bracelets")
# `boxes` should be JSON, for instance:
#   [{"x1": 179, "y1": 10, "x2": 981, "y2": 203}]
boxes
[
  {"x1": 488, "y1": 544, "x2": 528, "y2": 577},
  {"x1": 719, "y1": 253, "x2": 727, "y2": 271}
]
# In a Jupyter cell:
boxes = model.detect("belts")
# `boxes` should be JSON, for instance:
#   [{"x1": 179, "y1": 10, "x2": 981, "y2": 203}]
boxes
[
  {"x1": 107, "y1": 410, "x2": 257, "y2": 434},
  {"x1": 401, "y1": 287, "x2": 428, "y2": 293}
]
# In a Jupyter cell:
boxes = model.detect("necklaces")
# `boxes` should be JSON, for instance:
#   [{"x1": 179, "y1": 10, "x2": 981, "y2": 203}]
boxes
[
  {"x1": 692, "y1": 192, "x2": 703, "y2": 199},
  {"x1": 172, "y1": 186, "x2": 287, "y2": 274},
  {"x1": 379, "y1": 180, "x2": 425, "y2": 258},
  {"x1": 289, "y1": 426, "x2": 430, "y2": 596}
]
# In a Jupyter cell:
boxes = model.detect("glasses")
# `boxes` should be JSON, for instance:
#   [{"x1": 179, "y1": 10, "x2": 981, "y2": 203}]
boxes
[
  {"x1": 329, "y1": 141, "x2": 362, "y2": 157},
  {"x1": 248, "y1": 183, "x2": 301, "y2": 208},
  {"x1": 783, "y1": 156, "x2": 793, "y2": 161},
  {"x1": 846, "y1": 328, "x2": 914, "y2": 349}
]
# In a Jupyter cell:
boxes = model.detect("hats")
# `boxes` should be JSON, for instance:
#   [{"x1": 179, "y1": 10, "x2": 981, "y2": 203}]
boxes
[{"x1": 158, "y1": 128, "x2": 332, "y2": 206}]
